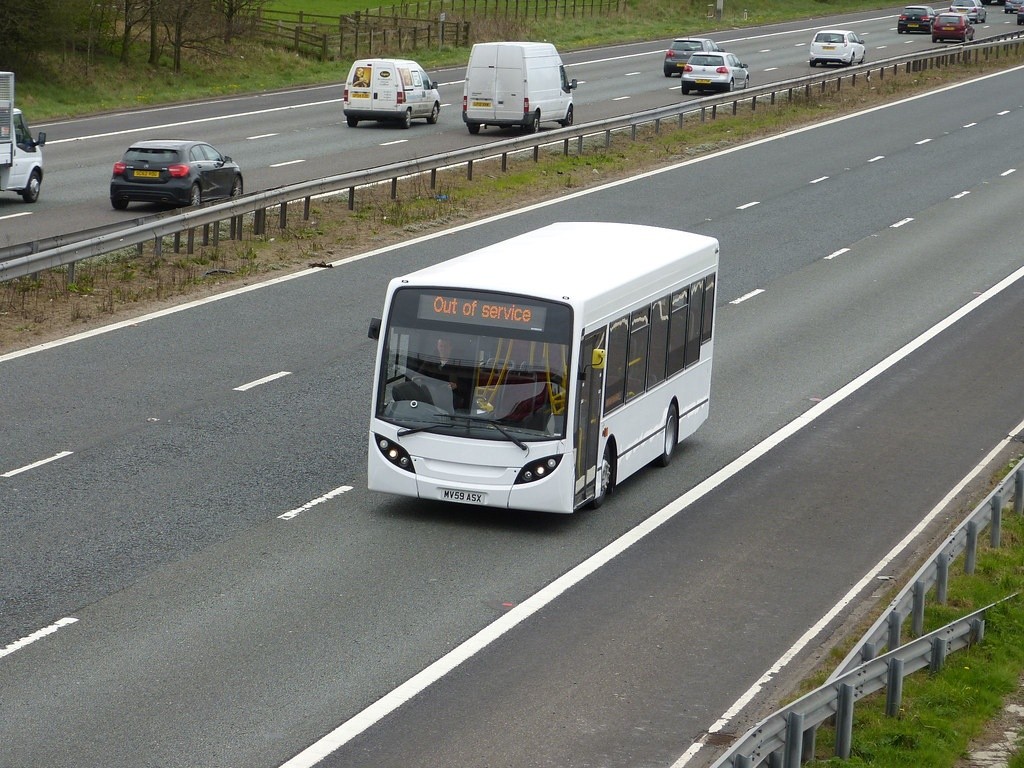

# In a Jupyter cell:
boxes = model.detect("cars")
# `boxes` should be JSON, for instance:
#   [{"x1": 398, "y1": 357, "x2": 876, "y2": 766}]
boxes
[
  {"x1": 1004, "y1": 0, "x2": 1023, "y2": 13},
  {"x1": 929, "y1": 12, "x2": 978, "y2": 44},
  {"x1": 949, "y1": 0, "x2": 986, "y2": 24},
  {"x1": 898, "y1": 5, "x2": 937, "y2": 32},
  {"x1": 809, "y1": 29, "x2": 865, "y2": 64},
  {"x1": 663, "y1": 35, "x2": 724, "y2": 79},
  {"x1": 1016, "y1": 1, "x2": 1024, "y2": 26},
  {"x1": 680, "y1": 51, "x2": 750, "y2": 95},
  {"x1": 109, "y1": 139, "x2": 245, "y2": 210}
]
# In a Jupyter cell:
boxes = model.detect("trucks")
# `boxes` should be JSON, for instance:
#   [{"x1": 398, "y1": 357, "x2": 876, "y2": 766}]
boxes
[{"x1": 0, "y1": 71, "x2": 47, "y2": 204}]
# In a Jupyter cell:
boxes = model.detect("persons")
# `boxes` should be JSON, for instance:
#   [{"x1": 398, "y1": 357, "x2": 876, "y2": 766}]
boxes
[{"x1": 414, "y1": 334, "x2": 474, "y2": 414}]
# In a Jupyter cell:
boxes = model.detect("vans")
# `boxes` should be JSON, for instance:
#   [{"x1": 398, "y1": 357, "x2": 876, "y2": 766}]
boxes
[
  {"x1": 463, "y1": 40, "x2": 579, "y2": 133},
  {"x1": 342, "y1": 58, "x2": 441, "y2": 127}
]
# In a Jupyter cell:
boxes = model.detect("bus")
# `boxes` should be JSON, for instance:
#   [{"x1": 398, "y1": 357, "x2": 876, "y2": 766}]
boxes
[{"x1": 362, "y1": 218, "x2": 719, "y2": 514}]
